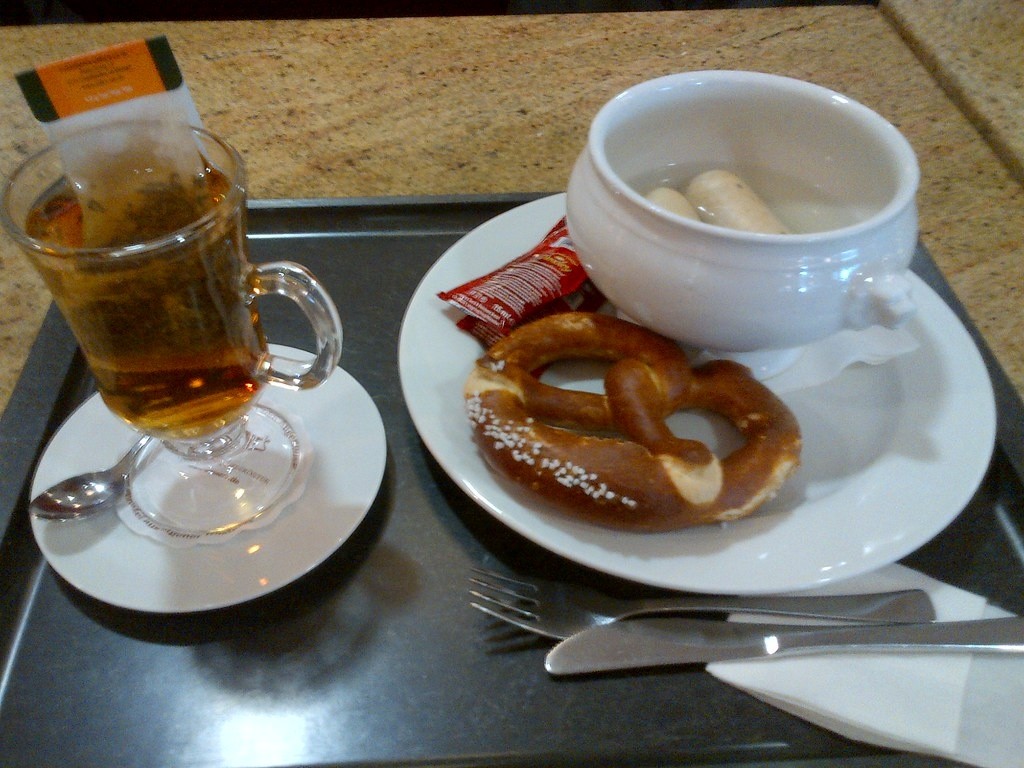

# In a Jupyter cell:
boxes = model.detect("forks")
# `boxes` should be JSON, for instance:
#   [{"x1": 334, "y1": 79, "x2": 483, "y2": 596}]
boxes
[{"x1": 468, "y1": 565, "x2": 936, "y2": 637}]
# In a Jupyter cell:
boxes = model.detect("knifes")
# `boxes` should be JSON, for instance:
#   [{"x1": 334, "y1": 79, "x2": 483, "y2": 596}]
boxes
[{"x1": 545, "y1": 615, "x2": 1024, "y2": 676}]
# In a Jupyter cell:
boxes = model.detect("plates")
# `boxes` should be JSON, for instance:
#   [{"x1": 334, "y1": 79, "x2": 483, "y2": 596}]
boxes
[
  {"x1": 29, "y1": 344, "x2": 387, "y2": 613},
  {"x1": 400, "y1": 193, "x2": 999, "y2": 597}
]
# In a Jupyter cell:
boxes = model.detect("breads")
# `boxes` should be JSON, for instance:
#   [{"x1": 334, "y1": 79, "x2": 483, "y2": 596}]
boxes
[{"x1": 469, "y1": 315, "x2": 803, "y2": 531}]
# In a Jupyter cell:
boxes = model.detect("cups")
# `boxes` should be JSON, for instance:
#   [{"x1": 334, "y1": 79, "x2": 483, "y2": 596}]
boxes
[{"x1": 8, "y1": 123, "x2": 343, "y2": 536}]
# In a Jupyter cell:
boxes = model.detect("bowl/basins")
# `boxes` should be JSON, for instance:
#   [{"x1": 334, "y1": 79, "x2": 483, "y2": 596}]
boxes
[{"x1": 569, "y1": 72, "x2": 921, "y2": 376}]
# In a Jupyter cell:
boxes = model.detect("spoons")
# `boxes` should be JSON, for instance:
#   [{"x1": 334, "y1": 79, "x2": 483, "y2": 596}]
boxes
[{"x1": 29, "y1": 431, "x2": 152, "y2": 519}]
